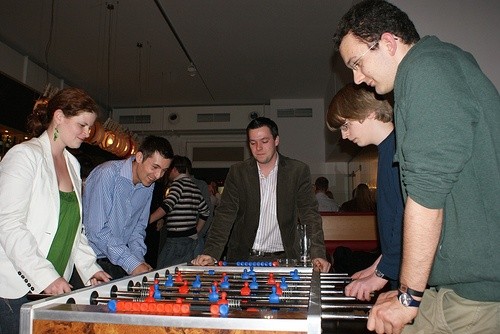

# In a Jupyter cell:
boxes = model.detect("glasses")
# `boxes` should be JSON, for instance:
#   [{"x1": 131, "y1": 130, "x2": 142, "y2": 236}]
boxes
[
  {"x1": 351, "y1": 41, "x2": 380, "y2": 71},
  {"x1": 339, "y1": 120, "x2": 350, "y2": 132}
]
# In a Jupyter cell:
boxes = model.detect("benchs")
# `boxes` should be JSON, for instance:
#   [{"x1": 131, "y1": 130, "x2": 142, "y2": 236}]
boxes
[{"x1": 318, "y1": 210, "x2": 380, "y2": 266}]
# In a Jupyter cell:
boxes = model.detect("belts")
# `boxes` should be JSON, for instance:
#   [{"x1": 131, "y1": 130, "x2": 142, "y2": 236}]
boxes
[{"x1": 249, "y1": 249, "x2": 285, "y2": 256}]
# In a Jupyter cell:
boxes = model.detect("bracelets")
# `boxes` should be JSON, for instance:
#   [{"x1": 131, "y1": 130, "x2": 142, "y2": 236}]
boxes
[{"x1": 397, "y1": 283, "x2": 424, "y2": 297}]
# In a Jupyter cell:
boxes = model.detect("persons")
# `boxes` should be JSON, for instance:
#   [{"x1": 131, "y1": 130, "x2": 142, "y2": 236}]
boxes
[
  {"x1": 208, "y1": 182, "x2": 221, "y2": 200},
  {"x1": 330, "y1": 0, "x2": 499, "y2": 333},
  {"x1": 0, "y1": 85, "x2": 112, "y2": 334},
  {"x1": 338, "y1": 183, "x2": 378, "y2": 211},
  {"x1": 324, "y1": 80, "x2": 405, "y2": 301},
  {"x1": 84, "y1": 135, "x2": 172, "y2": 273},
  {"x1": 169, "y1": 155, "x2": 207, "y2": 257},
  {"x1": 314, "y1": 176, "x2": 339, "y2": 212},
  {"x1": 147, "y1": 154, "x2": 211, "y2": 271},
  {"x1": 192, "y1": 116, "x2": 331, "y2": 271}
]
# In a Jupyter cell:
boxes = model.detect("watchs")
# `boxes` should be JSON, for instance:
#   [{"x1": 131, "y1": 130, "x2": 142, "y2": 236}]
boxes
[
  {"x1": 374, "y1": 269, "x2": 393, "y2": 282},
  {"x1": 397, "y1": 288, "x2": 421, "y2": 307}
]
